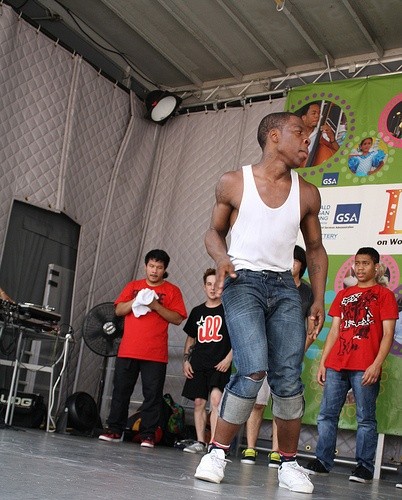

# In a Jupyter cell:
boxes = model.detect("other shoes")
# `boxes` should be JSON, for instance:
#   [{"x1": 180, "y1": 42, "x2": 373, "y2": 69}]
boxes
[
  {"x1": 99, "y1": 428, "x2": 124, "y2": 442},
  {"x1": 348, "y1": 465, "x2": 373, "y2": 484},
  {"x1": 304, "y1": 459, "x2": 329, "y2": 476},
  {"x1": 396, "y1": 480, "x2": 402, "y2": 488},
  {"x1": 140, "y1": 434, "x2": 154, "y2": 448},
  {"x1": 268, "y1": 452, "x2": 281, "y2": 468},
  {"x1": 241, "y1": 449, "x2": 257, "y2": 464}
]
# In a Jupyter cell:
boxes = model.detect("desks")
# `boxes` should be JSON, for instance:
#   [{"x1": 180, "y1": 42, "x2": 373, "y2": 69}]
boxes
[{"x1": 0, "y1": 322, "x2": 76, "y2": 432}]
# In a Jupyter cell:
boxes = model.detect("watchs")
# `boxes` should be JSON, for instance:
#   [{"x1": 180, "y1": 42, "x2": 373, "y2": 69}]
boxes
[{"x1": 183, "y1": 353, "x2": 189, "y2": 360}]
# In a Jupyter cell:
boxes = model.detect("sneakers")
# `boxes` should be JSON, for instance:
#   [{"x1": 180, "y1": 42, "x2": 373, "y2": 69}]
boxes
[
  {"x1": 277, "y1": 461, "x2": 315, "y2": 493},
  {"x1": 182, "y1": 441, "x2": 208, "y2": 454},
  {"x1": 194, "y1": 448, "x2": 232, "y2": 484}
]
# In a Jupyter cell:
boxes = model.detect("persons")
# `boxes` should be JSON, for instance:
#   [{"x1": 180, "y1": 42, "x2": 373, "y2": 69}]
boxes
[
  {"x1": 99, "y1": 249, "x2": 188, "y2": 448},
  {"x1": 348, "y1": 136, "x2": 386, "y2": 178},
  {"x1": 301, "y1": 103, "x2": 340, "y2": 168},
  {"x1": 241, "y1": 244, "x2": 313, "y2": 469},
  {"x1": 304, "y1": 247, "x2": 399, "y2": 485},
  {"x1": 194, "y1": 112, "x2": 329, "y2": 493},
  {"x1": 182, "y1": 268, "x2": 233, "y2": 453}
]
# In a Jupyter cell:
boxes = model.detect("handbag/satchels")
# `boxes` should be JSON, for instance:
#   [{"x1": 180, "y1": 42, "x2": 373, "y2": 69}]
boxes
[{"x1": 162, "y1": 393, "x2": 185, "y2": 436}]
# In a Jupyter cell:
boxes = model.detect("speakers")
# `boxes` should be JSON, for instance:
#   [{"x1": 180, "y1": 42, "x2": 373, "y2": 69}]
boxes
[{"x1": 0, "y1": 388, "x2": 47, "y2": 429}]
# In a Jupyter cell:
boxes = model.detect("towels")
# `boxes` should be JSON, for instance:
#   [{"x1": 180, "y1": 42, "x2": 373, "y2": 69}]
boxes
[{"x1": 131, "y1": 288, "x2": 159, "y2": 318}]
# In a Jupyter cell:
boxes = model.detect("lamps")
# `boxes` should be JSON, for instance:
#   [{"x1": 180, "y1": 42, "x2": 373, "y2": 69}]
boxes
[{"x1": 143, "y1": 89, "x2": 181, "y2": 125}]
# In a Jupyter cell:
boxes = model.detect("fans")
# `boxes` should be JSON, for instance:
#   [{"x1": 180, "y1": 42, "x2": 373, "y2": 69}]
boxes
[{"x1": 81, "y1": 302, "x2": 123, "y2": 417}]
